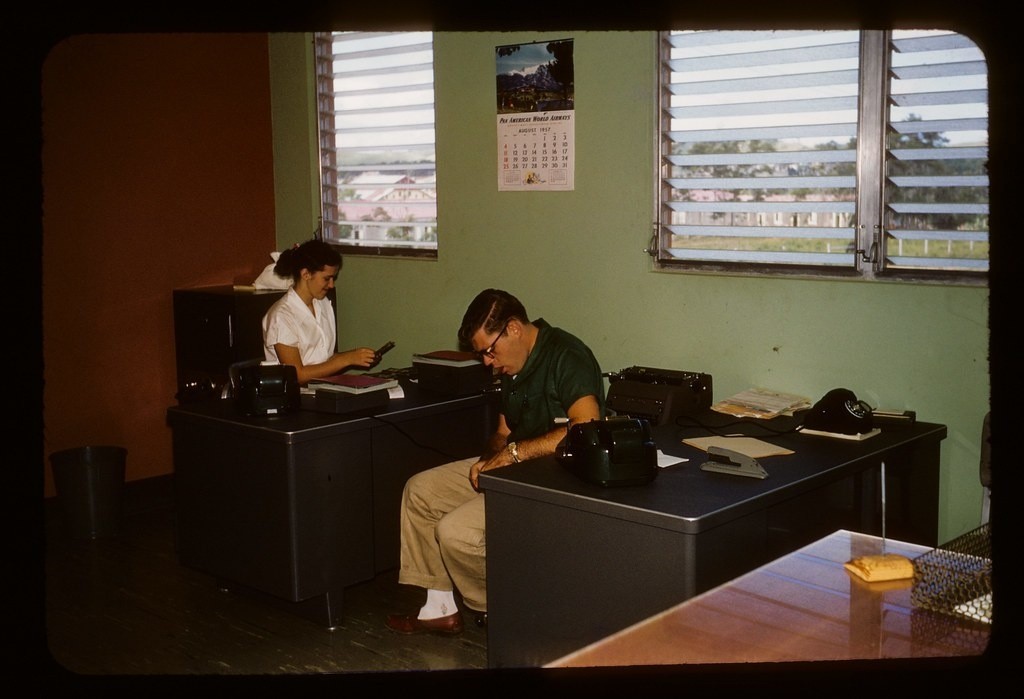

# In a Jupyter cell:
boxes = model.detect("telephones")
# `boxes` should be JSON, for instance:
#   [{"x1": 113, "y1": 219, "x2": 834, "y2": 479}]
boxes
[
  {"x1": 803, "y1": 388, "x2": 875, "y2": 436},
  {"x1": 556, "y1": 418, "x2": 657, "y2": 488}
]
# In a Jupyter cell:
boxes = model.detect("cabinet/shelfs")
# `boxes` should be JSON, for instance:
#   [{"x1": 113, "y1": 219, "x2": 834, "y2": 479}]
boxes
[{"x1": 174, "y1": 280, "x2": 335, "y2": 404}]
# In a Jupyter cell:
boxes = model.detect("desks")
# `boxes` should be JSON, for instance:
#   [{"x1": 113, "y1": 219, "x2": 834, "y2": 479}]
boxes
[
  {"x1": 542, "y1": 529, "x2": 992, "y2": 670},
  {"x1": 479, "y1": 406, "x2": 947, "y2": 668},
  {"x1": 167, "y1": 364, "x2": 495, "y2": 632}
]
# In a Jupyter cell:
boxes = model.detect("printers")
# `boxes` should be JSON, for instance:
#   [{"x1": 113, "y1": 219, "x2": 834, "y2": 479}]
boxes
[{"x1": 604, "y1": 366, "x2": 715, "y2": 428}]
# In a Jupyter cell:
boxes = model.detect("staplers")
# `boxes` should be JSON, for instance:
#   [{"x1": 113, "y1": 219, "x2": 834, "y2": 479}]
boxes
[{"x1": 699, "y1": 445, "x2": 769, "y2": 479}]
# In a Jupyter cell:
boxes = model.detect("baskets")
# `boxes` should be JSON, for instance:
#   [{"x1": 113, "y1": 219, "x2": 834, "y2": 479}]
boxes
[{"x1": 910, "y1": 523, "x2": 991, "y2": 622}]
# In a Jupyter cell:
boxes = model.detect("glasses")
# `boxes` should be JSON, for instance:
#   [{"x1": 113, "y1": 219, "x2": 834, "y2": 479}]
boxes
[{"x1": 473, "y1": 321, "x2": 511, "y2": 363}]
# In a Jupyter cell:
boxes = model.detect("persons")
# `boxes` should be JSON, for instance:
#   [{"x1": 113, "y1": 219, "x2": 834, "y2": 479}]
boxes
[
  {"x1": 262, "y1": 240, "x2": 374, "y2": 386},
  {"x1": 383, "y1": 288, "x2": 606, "y2": 637}
]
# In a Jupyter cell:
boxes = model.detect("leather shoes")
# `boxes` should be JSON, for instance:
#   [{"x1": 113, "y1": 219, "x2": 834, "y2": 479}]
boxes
[{"x1": 384, "y1": 611, "x2": 464, "y2": 638}]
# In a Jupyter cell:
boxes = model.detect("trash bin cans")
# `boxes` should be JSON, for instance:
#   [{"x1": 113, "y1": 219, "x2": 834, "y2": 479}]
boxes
[{"x1": 52, "y1": 445, "x2": 127, "y2": 543}]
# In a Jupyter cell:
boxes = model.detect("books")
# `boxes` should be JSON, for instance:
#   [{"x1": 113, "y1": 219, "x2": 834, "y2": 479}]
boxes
[
  {"x1": 308, "y1": 372, "x2": 398, "y2": 395},
  {"x1": 412, "y1": 350, "x2": 484, "y2": 368}
]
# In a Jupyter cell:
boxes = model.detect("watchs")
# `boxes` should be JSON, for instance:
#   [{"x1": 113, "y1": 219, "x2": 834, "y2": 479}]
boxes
[{"x1": 507, "y1": 442, "x2": 521, "y2": 463}]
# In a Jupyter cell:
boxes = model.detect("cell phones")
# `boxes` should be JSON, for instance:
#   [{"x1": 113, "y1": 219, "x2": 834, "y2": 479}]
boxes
[{"x1": 375, "y1": 341, "x2": 395, "y2": 357}]
